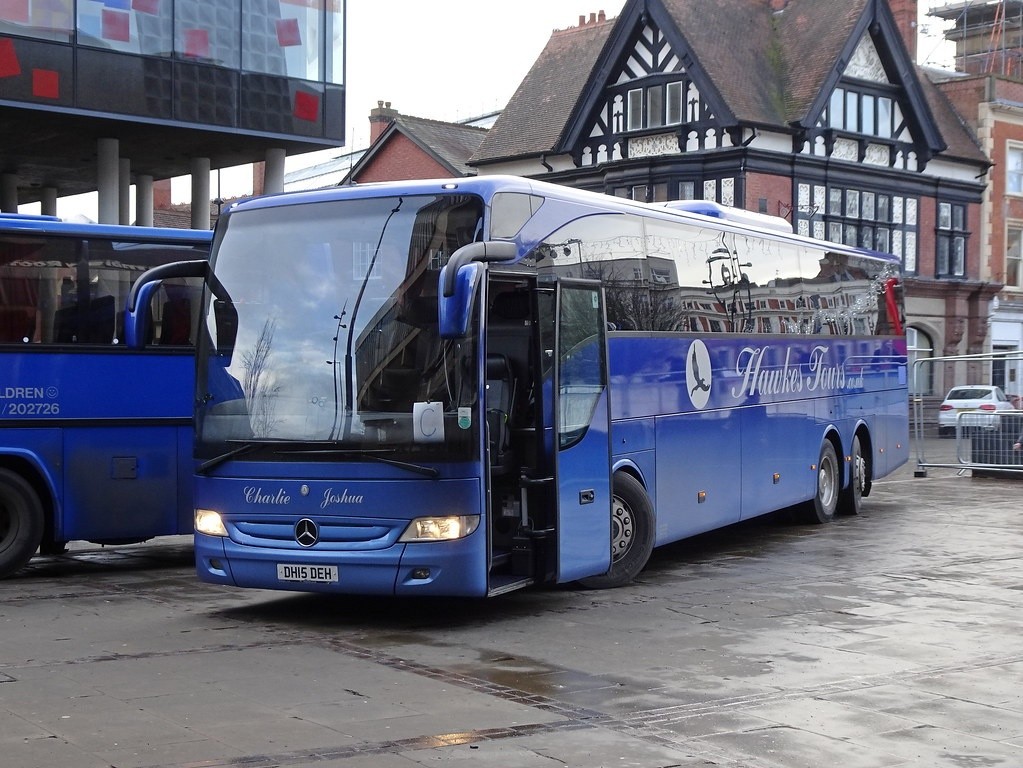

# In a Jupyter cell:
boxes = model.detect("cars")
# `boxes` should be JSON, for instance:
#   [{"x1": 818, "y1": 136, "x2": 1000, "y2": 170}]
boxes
[{"x1": 937, "y1": 385, "x2": 1016, "y2": 434}]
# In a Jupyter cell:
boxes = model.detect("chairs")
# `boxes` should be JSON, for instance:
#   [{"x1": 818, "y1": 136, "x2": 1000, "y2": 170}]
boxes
[
  {"x1": 0, "y1": 275, "x2": 193, "y2": 348},
  {"x1": 467, "y1": 353, "x2": 515, "y2": 476}
]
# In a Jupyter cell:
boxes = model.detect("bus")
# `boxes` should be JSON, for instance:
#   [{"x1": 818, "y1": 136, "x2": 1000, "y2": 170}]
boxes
[
  {"x1": 125, "y1": 170, "x2": 915, "y2": 607},
  {"x1": 0, "y1": 212, "x2": 338, "y2": 579}
]
